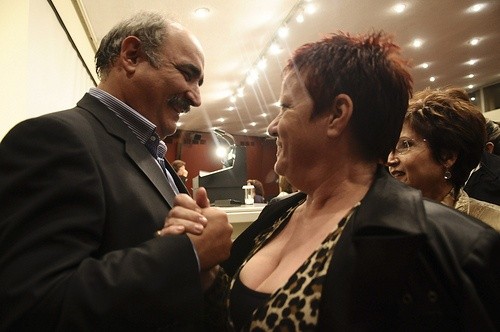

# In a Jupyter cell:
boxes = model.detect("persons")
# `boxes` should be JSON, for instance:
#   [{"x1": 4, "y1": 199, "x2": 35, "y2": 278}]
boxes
[
  {"x1": 171, "y1": 160, "x2": 189, "y2": 183},
  {"x1": 246, "y1": 177, "x2": 266, "y2": 204},
  {"x1": 0, "y1": 13, "x2": 232, "y2": 331},
  {"x1": 160, "y1": 34, "x2": 499, "y2": 332},
  {"x1": 385, "y1": 86, "x2": 500, "y2": 229},
  {"x1": 270, "y1": 174, "x2": 302, "y2": 203}
]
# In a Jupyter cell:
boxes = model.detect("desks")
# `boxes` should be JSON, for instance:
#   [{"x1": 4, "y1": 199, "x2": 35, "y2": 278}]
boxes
[{"x1": 211, "y1": 202, "x2": 267, "y2": 243}]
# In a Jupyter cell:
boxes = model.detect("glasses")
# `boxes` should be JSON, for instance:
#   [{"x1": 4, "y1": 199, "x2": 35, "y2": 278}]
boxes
[{"x1": 391, "y1": 138, "x2": 432, "y2": 153}]
